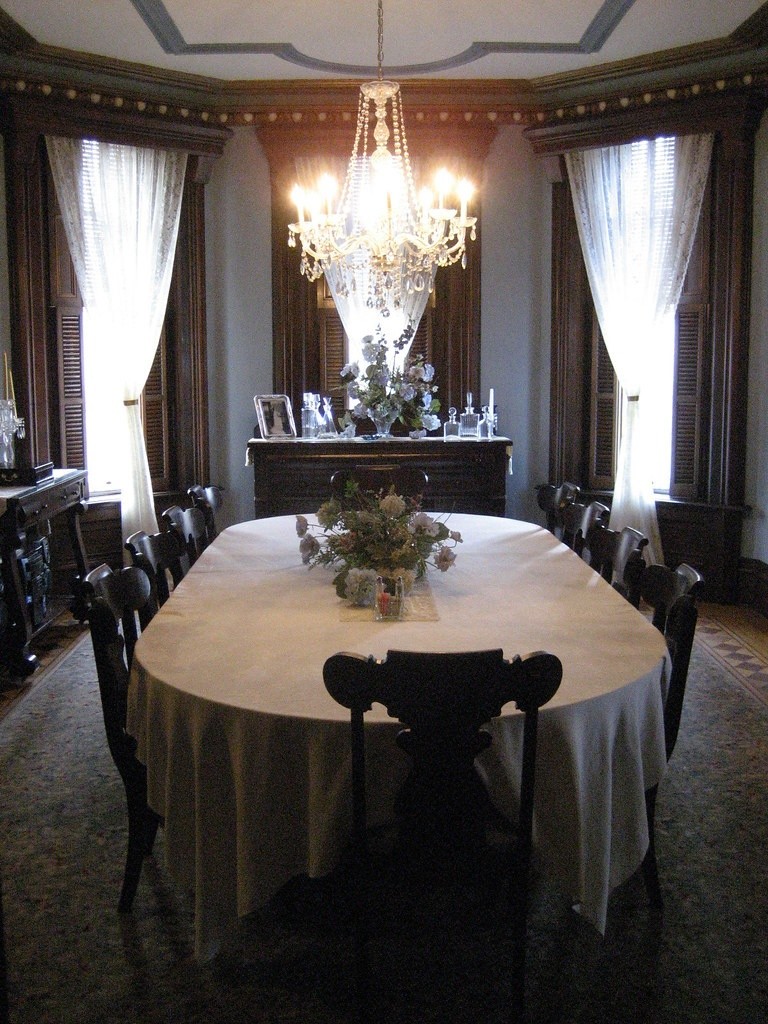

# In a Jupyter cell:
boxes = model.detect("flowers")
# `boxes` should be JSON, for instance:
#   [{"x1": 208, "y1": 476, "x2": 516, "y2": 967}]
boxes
[
  {"x1": 336, "y1": 341, "x2": 440, "y2": 429},
  {"x1": 297, "y1": 483, "x2": 463, "y2": 608}
]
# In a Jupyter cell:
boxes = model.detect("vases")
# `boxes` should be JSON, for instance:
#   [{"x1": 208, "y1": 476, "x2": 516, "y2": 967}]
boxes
[{"x1": 371, "y1": 416, "x2": 395, "y2": 438}]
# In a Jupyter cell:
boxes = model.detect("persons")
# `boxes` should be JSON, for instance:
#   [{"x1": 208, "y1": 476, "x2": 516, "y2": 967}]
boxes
[{"x1": 270, "y1": 406, "x2": 285, "y2": 433}]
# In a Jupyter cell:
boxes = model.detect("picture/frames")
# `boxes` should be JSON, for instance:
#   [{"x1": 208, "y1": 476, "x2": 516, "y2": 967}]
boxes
[{"x1": 252, "y1": 393, "x2": 299, "y2": 440}]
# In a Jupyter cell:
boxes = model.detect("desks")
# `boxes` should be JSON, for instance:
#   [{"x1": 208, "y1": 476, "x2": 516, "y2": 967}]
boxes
[
  {"x1": 125, "y1": 511, "x2": 674, "y2": 963},
  {"x1": 0, "y1": 469, "x2": 88, "y2": 681},
  {"x1": 247, "y1": 437, "x2": 513, "y2": 520}
]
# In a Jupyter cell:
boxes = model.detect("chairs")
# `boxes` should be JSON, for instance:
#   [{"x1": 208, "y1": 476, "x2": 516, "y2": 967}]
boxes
[
  {"x1": 72, "y1": 484, "x2": 224, "y2": 915},
  {"x1": 552, "y1": 482, "x2": 705, "y2": 911},
  {"x1": 324, "y1": 648, "x2": 562, "y2": 1024}
]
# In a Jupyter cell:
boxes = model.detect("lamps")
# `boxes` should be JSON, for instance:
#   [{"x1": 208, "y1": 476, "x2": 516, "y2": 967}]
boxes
[{"x1": 287, "y1": 1, "x2": 476, "y2": 313}]
